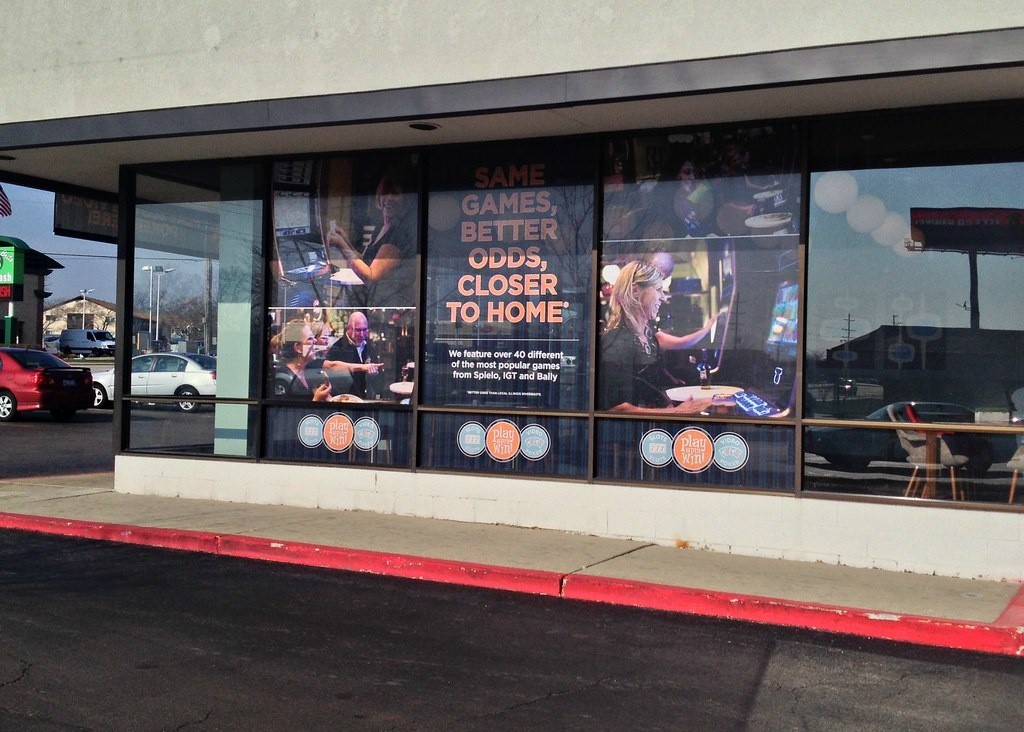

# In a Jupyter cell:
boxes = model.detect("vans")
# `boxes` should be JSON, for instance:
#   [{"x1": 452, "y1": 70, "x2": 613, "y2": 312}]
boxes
[{"x1": 60, "y1": 328, "x2": 116, "y2": 358}]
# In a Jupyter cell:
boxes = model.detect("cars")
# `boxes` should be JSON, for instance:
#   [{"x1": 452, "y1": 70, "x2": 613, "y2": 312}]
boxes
[
  {"x1": 90, "y1": 352, "x2": 221, "y2": 411},
  {"x1": 805, "y1": 401, "x2": 1018, "y2": 476},
  {"x1": 43, "y1": 335, "x2": 59, "y2": 350},
  {"x1": 833, "y1": 378, "x2": 860, "y2": 394},
  {"x1": 0, "y1": 345, "x2": 93, "y2": 423}
]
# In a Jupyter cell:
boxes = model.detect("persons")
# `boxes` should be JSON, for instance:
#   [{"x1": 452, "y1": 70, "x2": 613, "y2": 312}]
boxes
[
  {"x1": 326, "y1": 174, "x2": 416, "y2": 305},
  {"x1": 266, "y1": 311, "x2": 412, "y2": 404},
  {"x1": 641, "y1": 135, "x2": 801, "y2": 238},
  {"x1": 598, "y1": 260, "x2": 721, "y2": 416}
]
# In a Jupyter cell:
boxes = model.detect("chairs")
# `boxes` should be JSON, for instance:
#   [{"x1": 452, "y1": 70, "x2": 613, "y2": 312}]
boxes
[{"x1": 887, "y1": 403, "x2": 1024, "y2": 506}]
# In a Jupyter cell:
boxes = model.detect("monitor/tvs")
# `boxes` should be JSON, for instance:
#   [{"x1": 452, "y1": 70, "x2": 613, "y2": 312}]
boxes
[{"x1": 765, "y1": 283, "x2": 798, "y2": 357}]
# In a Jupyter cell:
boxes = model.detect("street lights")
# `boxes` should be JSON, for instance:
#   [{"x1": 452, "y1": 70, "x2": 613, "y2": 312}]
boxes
[
  {"x1": 154, "y1": 267, "x2": 178, "y2": 342},
  {"x1": 79, "y1": 288, "x2": 97, "y2": 331},
  {"x1": 142, "y1": 264, "x2": 162, "y2": 340}
]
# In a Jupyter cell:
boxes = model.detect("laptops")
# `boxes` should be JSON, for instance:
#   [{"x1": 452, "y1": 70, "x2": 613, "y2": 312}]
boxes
[{"x1": 276, "y1": 226, "x2": 341, "y2": 284}]
